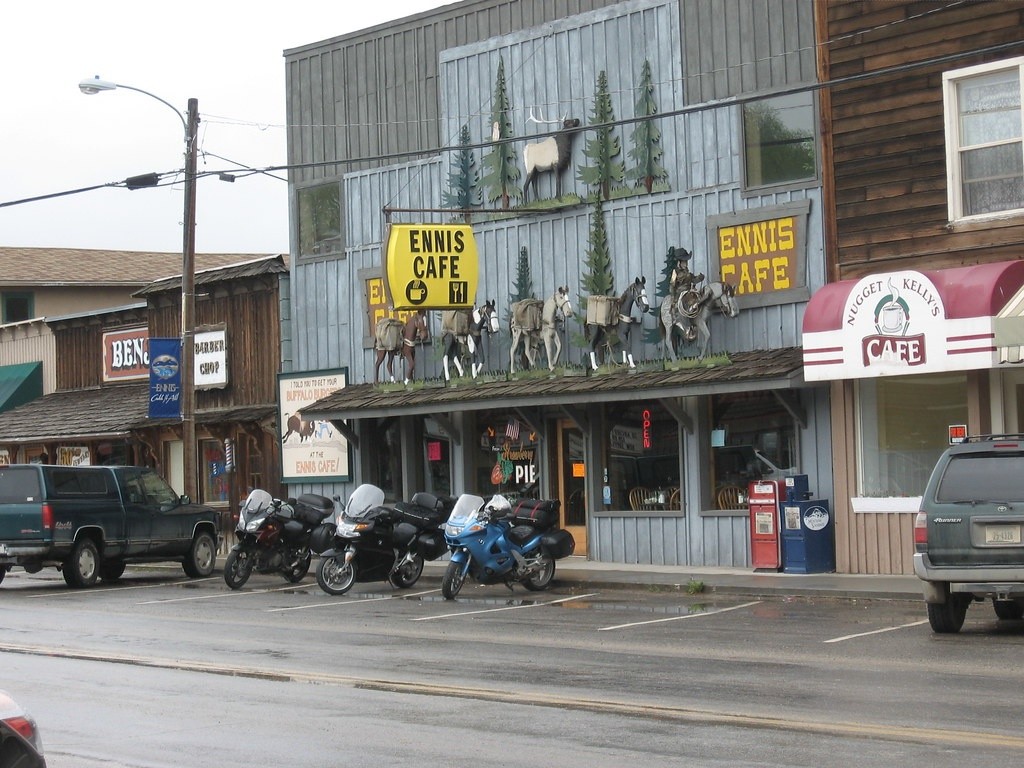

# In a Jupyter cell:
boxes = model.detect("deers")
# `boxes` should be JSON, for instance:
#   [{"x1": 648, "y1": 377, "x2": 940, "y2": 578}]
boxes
[{"x1": 519, "y1": 105, "x2": 581, "y2": 203}]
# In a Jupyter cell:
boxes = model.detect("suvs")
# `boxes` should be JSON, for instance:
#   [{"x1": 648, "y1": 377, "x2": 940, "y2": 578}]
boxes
[
  {"x1": 911, "y1": 432, "x2": 1024, "y2": 634},
  {"x1": 0, "y1": 462, "x2": 225, "y2": 589}
]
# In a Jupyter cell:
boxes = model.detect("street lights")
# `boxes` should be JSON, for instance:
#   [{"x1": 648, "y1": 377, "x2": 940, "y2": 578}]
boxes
[{"x1": 77, "y1": 76, "x2": 201, "y2": 504}]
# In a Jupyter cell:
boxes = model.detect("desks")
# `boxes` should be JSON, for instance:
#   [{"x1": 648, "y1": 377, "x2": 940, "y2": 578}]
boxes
[
  {"x1": 728, "y1": 502, "x2": 748, "y2": 509},
  {"x1": 639, "y1": 503, "x2": 674, "y2": 510}
]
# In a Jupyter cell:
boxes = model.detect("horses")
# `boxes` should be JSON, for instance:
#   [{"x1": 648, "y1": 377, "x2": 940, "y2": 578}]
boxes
[{"x1": 372, "y1": 275, "x2": 741, "y2": 388}]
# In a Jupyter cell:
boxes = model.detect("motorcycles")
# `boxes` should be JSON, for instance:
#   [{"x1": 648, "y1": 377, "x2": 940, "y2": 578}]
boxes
[
  {"x1": 315, "y1": 484, "x2": 459, "y2": 596},
  {"x1": 224, "y1": 485, "x2": 335, "y2": 590},
  {"x1": 442, "y1": 493, "x2": 575, "y2": 601}
]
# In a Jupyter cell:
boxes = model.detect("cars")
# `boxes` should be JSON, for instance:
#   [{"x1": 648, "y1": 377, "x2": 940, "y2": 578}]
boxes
[
  {"x1": 622, "y1": 444, "x2": 798, "y2": 510},
  {"x1": 869, "y1": 448, "x2": 941, "y2": 498}
]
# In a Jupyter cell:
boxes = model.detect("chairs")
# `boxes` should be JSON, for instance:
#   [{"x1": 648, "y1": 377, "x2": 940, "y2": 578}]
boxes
[
  {"x1": 717, "y1": 485, "x2": 746, "y2": 509},
  {"x1": 630, "y1": 487, "x2": 680, "y2": 511}
]
